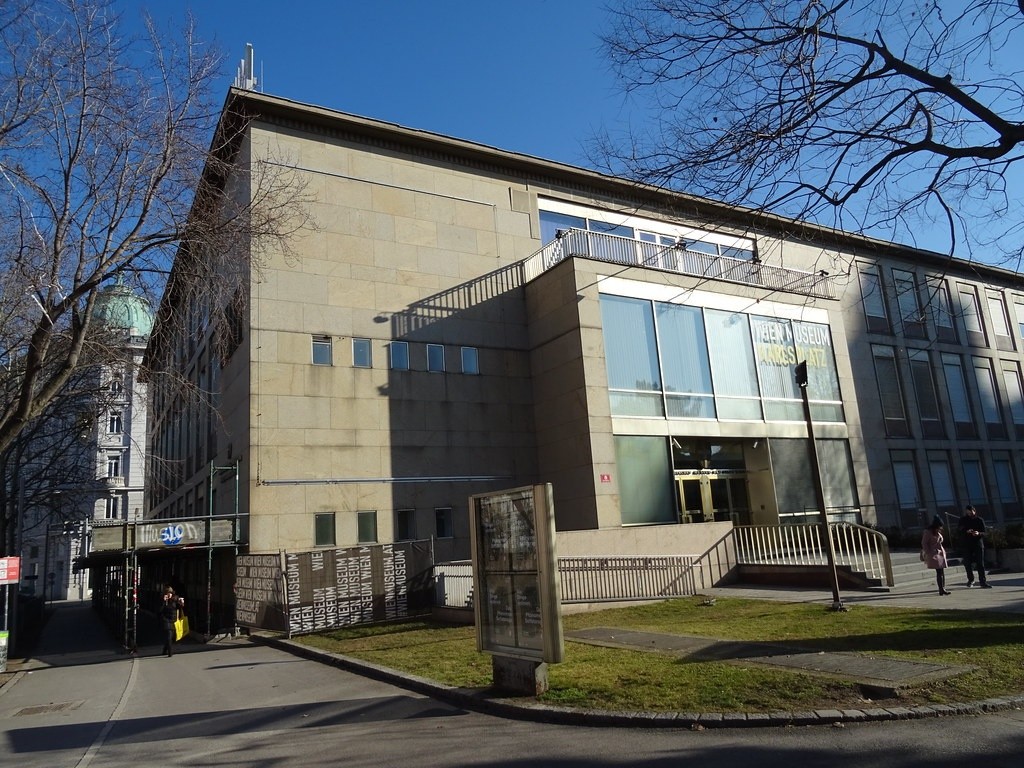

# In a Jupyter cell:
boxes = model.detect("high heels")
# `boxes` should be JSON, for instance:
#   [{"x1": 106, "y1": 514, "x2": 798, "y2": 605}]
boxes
[{"x1": 939, "y1": 588, "x2": 951, "y2": 596}]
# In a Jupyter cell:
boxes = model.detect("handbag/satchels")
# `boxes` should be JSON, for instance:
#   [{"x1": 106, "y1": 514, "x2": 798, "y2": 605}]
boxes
[
  {"x1": 920, "y1": 548, "x2": 925, "y2": 561},
  {"x1": 174, "y1": 609, "x2": 189, "y2": 641}
]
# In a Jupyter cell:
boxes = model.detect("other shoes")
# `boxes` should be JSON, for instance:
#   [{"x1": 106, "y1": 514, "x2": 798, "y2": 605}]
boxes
[
  {"x1": 980, "y1": 582, "x2": 992, "y2": 589},
  {"x1": 967, "y1": 579, "x2": 975, "y2": 588}
]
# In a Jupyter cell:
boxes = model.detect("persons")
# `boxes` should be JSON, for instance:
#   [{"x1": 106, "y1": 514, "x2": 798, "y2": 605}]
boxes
[
  {"x1": 957, "y1": 505, "x2": 992, "y2": 588},
  {"x1": 919, "y1": 513, "x2": 952, "y2": 596},
  {"x1": 158, "y1": 586, "x2": 185, "y2": 658}
]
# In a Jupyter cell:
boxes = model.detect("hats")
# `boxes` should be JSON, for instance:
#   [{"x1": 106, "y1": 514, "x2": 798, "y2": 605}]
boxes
[{"x1": 165, "y1": 586, "x2": 175, "y2": 595}]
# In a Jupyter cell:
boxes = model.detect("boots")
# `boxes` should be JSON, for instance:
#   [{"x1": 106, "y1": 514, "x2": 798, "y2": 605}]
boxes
[
  {"x1": 162, "y1": 640, "x2": 167, "y2": 655},
  {"x1": 168, "y1": 639, "x2": 172, "y2": 657}
]
